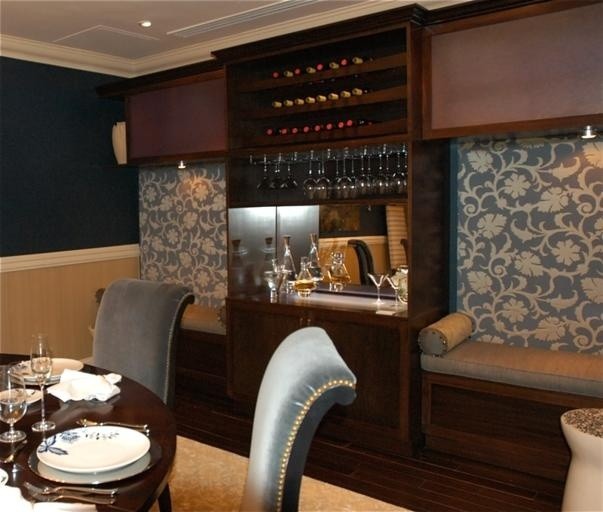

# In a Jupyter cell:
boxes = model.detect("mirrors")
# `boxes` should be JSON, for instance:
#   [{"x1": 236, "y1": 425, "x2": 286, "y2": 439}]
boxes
[{"x1": 275, "y1": 204, "x2": 408, "y2": 301}]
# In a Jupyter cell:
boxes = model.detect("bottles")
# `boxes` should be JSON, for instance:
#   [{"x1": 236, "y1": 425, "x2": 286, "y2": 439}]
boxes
[
  {"x1": 272, "y1": 233, "x2": 316, "y2": 298},
  {"x1": 229, "y1": 238, "x2": 249, "y2": 294},
  {"x1": 307, "y1": 234, "x2": 351, "y2": 293},
  {"x1": 257, "y1": 237, "x2": 274, "y2": 295},
  {"x1": 264, "y1": 57, "x2": 377, "y2": 135}
]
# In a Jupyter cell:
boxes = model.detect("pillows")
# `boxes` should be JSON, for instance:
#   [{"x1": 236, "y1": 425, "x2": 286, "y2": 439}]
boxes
[{"x1": 417, "y1": 313, "x2": 477, "y2": 356}]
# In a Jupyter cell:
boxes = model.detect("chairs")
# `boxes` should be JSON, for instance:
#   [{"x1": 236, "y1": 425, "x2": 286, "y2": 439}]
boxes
[
  {"x1": 346, "y1": 239, "x2": 376, "y2": 287},
  {"x1": 238, "y1": 326, "x2": 357, "y2": 512},
  {"x1": 93, "y1": 279, "x2": 196, "y2": 405}
]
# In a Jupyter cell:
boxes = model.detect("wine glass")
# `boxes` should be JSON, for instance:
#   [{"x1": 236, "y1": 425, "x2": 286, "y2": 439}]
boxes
[
  {"x1": 264, "y1": 272, "x2": 285, "y2": 303},
  {"x1": 256, "y1": 145, "x2": 407, "y2": 200},
  {"x1": 369, "y1": 273, "x2": 403, "y2": 305},
  {"x1": 0, "y1": 334, "x2": 56, "y2": 443}
]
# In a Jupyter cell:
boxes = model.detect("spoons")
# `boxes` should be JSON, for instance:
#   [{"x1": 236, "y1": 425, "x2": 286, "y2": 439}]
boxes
[
  {"x1": 21, "y1": 482, "x2": 117, "y2": 505},
  {"x1": 75, "y1": 418, "x2": 148, "y2": 430}
]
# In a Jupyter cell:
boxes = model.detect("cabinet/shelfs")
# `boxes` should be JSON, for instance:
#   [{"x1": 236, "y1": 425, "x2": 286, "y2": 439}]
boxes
[
  {"x1": 216, "y1": 25, "x2": 410, "y2": 203},
  {"x1": 225, "y1": 303, "x2": 399, "y2": 434}
]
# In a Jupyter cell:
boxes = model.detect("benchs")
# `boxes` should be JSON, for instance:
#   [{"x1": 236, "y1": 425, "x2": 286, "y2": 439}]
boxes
[{"x1": 422, "y1": 341, "x2": 603, "y2": 484}]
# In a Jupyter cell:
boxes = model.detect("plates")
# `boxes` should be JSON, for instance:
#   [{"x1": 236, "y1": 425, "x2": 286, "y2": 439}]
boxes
[
  {"x1": 6, "y1": 357, "x2": 84, "y2": 382},
  {"x1": 37, "y1": 425, "x2": 151, "y2": 474}
]
